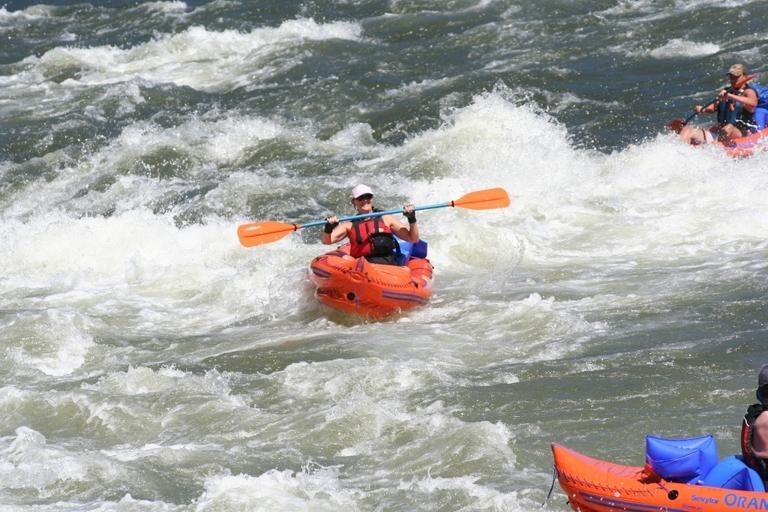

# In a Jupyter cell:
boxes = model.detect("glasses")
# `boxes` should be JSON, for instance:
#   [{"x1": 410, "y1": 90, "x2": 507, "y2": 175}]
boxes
[{"x1": 357, "y1": 196, "x2": 372, "y2": 201}]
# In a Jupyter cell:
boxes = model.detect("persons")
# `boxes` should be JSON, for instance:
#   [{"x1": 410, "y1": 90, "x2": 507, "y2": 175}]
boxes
[
  {"x1": 321, "y1": 183, "x2": 420, "y2": 265},
  {"x1": 680, "y1": 63, "x2": 759, "y2": 146},
  {"x1": 741, "y1": 365, "x2": 768, "y2": 489}
]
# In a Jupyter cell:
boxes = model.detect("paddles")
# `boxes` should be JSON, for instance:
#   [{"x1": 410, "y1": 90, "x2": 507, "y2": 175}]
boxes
[
  {"x1": 239, "y1": 188, "x2": 508, "y2": 247},
  {"x1": 670, "y1": 76, "x2": 755, "y2": 134}
]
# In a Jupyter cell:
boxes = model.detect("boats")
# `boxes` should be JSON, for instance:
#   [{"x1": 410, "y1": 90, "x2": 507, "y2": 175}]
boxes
[
  {"x1": 309, "y1": 235, "x2": 438, "y2": 323},
  {"x1": 665, "y1": 117, "x2": 765, "y2": 161},
  {"x1": 547, "y1": 442, "x2": 767, "y2": 511}
]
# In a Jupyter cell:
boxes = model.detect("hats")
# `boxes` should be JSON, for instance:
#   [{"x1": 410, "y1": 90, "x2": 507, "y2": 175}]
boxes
[
  {"x1": 352, "y1": 184, "x2": 373, "y2": 199},
  {"x1": 726, "y1": 64, "x2": 745, "y2": 77}
]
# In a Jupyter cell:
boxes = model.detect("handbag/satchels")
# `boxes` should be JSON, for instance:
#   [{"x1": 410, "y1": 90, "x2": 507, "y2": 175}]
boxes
[{"x1": 371, "y1": 232, "x2": 393, "y2": 256}]
